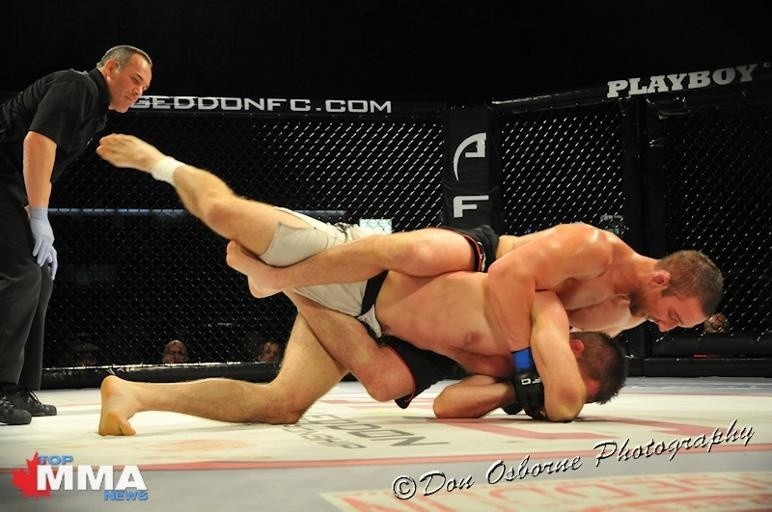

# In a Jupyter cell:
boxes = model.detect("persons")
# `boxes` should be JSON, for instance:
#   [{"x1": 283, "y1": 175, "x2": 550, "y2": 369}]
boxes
[
  {"x1": 256, "y1": 339, "x2": 281, "y2": 362},
  {"x1": 224, "y1": 221, "x2": 725, "y2": 410},
  {"x1": 0, "y1": 45, "x2": 155, "y2": 427},
  {"x1": 703, "y1": 311, "x2": 731, "y2": 333},
  {"x1": 95, "y1": 130, "x2": 628, "y2": 437},
  {"x1": 161, "y1": 339, "x2": 191, "y2": 363}
]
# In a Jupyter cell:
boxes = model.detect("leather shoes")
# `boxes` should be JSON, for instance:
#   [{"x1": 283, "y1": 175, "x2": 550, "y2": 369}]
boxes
[
  {"x1": 6, "y1": 387, "x2": 56, "y2": 416},
  {"x1": 0, "y1": 391, "x2": 32, "y2": 425}
]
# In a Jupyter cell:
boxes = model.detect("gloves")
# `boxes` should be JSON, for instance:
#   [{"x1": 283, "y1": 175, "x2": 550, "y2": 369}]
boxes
[
  {"x1": 29, "y1": 207, "x2": 55, "y2": 268},
  {"x1": 513, "y1": 366, "x2": 545, "y2": 410},
  {"x1": 47, "y1": 247, "x2": 58, "y2": 282}
]
